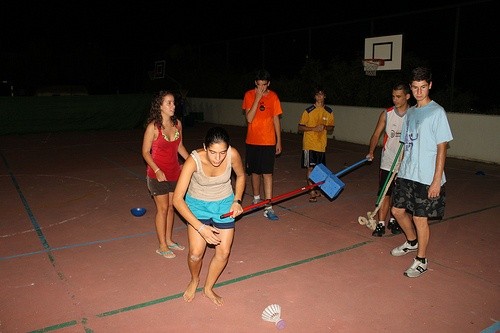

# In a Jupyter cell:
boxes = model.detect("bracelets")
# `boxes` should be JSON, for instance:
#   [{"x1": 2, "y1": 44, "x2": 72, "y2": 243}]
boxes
[
  {"x1": 197, "y1": 224, "x2": 205, "y2": 232},
  {"x1": 154, "y1": 168, "x2": 160, "y2": 173}
]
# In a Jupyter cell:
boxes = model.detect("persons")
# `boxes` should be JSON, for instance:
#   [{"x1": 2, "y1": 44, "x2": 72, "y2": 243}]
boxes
[
  {"x1": 298, "y1": 88, "x2": 335, "y2": 203},
  {"x1": 390, "y1": 67, "x2": 454, "y2": 278},
  {"x1": 242, "y1": 72, "x2": 284, "y2": 220},
  {"x1": 172, "y1": 126, "x2": 247, "y2": 306},
  {"x1": 368, "y1": 81, "x2": 413, "y2": 238},
  {"x1": 142, "y1": 90, "x2": 190, "y2": 258}
]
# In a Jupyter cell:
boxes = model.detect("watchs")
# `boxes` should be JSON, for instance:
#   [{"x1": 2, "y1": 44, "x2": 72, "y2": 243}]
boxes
[{"x1": 233, "y1": 200, "x2": 241, "y2": 204}]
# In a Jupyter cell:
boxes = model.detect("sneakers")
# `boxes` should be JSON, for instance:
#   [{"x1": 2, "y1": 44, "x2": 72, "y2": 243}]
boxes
[
  {"x1": 372, "y1": 223, "x2": 385, "y2": 237},
  {"x1": 387, "y1": 220, "x2": 402, "y2": 234},
  {"x1": 390, "y1": 241, "x2": 418, "y2": 256},
  {"x1": 404, "y1": 258, "x2": 428, "y2": 278},
  {"x1": 253, "y1": 198, "x2": 261, "y2": 205},
  {"x1": 263, "y1": 208, "x2": 278, "y2": 219}
]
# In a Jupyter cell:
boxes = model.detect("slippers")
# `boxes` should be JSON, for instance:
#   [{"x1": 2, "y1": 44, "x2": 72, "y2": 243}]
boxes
[
  {"x1": 156, "y1": 249, "x2": 175, "y2": 259},
  {"x1": 169, "y1": 243, "x2": 185, "y2": 250},
  {"x1": 315, "y1": 192, "x2": 321, "y2": 197},
  {"x1": 310, "y1": 197, "x2": 317, "y2": 202}
]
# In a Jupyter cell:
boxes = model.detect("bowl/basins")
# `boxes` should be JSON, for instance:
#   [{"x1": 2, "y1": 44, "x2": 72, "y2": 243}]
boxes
[{"x1": 131, "y1": 208, "x2": 147, "y2": 216}]
